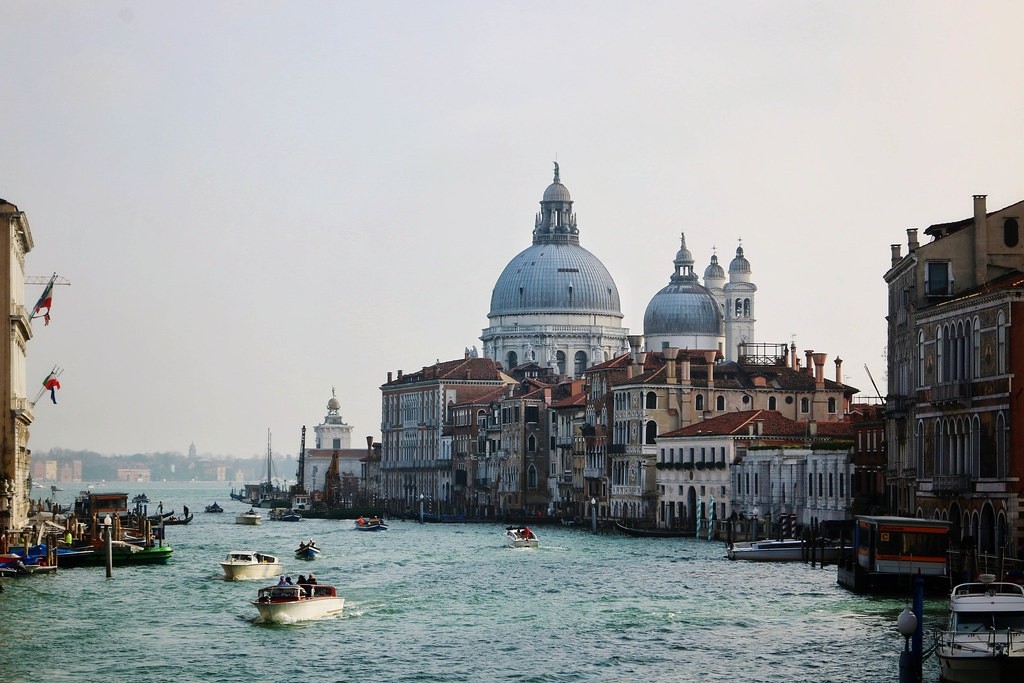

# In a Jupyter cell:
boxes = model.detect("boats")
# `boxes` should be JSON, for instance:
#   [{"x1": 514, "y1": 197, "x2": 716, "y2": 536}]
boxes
[
  {"x1": 241, "y1": 493, "x2": 277, "y2": 508},
  {"x1": 0, "y1": 492, "x2": 174, "y2": 577},
  {"x1": 236, "y1": 512, "x2": 262, "y2": 524},
  {"x1": 219, "y1": 550, "x2": 283, "y2": 579},
  {"x1": 837, "y1": 511, "x2": 955, "y2": 597},
  {"x1": 32, "y1": 482, "x2": 46, "y2": 488},
  {"x1": 934, "y1": 573, "x2": 1024, "y2": 683},
  {"x1": 501, "y1": 526, "x2": 540, "y2": 549},
  {"x1": 87, "y1": 483, "x2": 107, "y2": 488},
  {"x1": 51, "y1": 486, "x2": 63, "y2": 490},
  {"x1": 268, "y1": 511, "x2": 302, "y2": 521},
  {"x1": 250, "y1": 583, "x2": 345, "y2": 622},
  {"x1": 205, "y1": 506, "x2": 223, "y2": 512},
  {"x1": 290, "y1": 493, "x2": 315, "y2": 511},
  {"x1": 726, "y1": 538, "x2": 853, "y2": 562},
  {"x1": 150, "y1": 514, "x2": 193, "y2": 526},
  {"x1": 354, "y1": 517, "x2": 388, "y2": 531},
  {"x1": 294, "y1": 545, "x2": 320, "y2": 559}
]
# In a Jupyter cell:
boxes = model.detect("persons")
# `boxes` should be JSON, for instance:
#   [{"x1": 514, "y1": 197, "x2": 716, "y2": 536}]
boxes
[
  {"x1": 309, "y1": 540, "x2": 313, "y2": 546},
  {"x1": 278, "y1": 576, "x2": 295, "y2": 595},
  {"x1": 359, "y1": 516, "x2": 365, "y2": 524},
  {"x1": 65, "y1": 530, "x2": 72, "y2": 546},
  {"x1": 297, "y1": 574, "x2": 317, "y2": 597},
  {"x1": 183, "y1": 506, "x2": 189, "y2": 518},
  {"x1": 249, "y1": 508, "x2": 254, "y2": 514},
  {"x1": 379, "y1": 517, "x2": 384, "y2": 523},
  {"x1": 158, "y1": 501, "x2": 163, "y2": 514},
  {"x1": 517, "y1": 527, "x2": 529, "y2": 538},
  {"x1": 300, "y1": 541, "x2": 305, "y2": 547}
]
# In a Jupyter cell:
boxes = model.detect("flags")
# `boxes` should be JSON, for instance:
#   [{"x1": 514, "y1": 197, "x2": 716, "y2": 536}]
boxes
[
  {"x1": 35, "y1": 281, "x2": 53, "y2": 326},
  {"x1": 42, "y1": 371, "x2": 61, "y2": 404}
]
